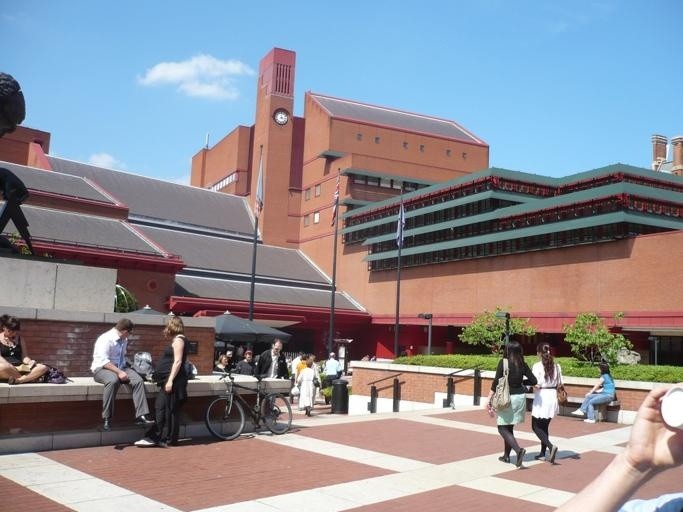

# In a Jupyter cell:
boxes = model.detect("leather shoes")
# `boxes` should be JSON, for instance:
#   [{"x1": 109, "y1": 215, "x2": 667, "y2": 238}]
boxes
[
  {"x1": 550, "y1": 446, "x2": 558, "y2": 463},
  {"x1": 136, "y1": 415, "x2": 154, "y2": 426},
  {"x1": 535, "y1": 456, "x2": 546, "y2": 461},
  {"x1": 104, "y1": 418, "x2": 110, "y2": 432},
  {"x1": 499, "y1": 457, "x2": 510, "y2": 462},
  {"x1": 516, "y1": 448, "x2": 526, "y2": 466}
]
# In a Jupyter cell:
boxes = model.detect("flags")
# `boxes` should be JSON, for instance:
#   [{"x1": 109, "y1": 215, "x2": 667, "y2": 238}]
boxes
[
  {"x1": 397, "y1": 197, "x2": 405, "y2": 248},
  {"x1": 330, "y1": 174, "x2": 341, "y2": 226},
  {"x1": 255, "y1": 158, "x2": 263, "y2": 217}
]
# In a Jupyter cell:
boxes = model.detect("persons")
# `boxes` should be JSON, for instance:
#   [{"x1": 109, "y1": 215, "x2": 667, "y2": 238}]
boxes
[
  {"x1": 0, "y1": 315, "x2": 48, "y2": 384},
  {"x1": 525, "y1": 342, "x2": 562, "y2": 463},
  {"x1": 361, "y1": 355, "x2": 370, "y2": 361},
  {"x1": 0, "y1": 72, "x2": 34, "y2": 257},
  {"x1": 134, "y1": 316, "x2": 187, "y2": 447},
  {"x1": 405, "y1": 344, "x2": 416, "y2": 357},
  {"x1": 370, "y1": 355, "x2": 375, "y2": 361},
  {"x1": 546, "y1": 384, "x2": 683, "y2": 511},
  {"x1": 487, "y1": 341, "x2": 537, "y2": 467},
  {"x1": 571, "y1": 362, "x2": 615, "y2": 422},
  {"x1": 90, "y1": 318, "x2": 155, "y2": 432},
  {"x1": 214, "y1": 339, "x2": 344, "y2": 416}
]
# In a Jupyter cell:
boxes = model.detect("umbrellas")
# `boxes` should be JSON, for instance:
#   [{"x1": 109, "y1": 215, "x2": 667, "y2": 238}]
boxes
[
  {"x1": 206, "y1": 311, "x2": 293, "y2": 349},
  {"x1": 125, "y1": 305, "x2": 166, "y2": 315}
]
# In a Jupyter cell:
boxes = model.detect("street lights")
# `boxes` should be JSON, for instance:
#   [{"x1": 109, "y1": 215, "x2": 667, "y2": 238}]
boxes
[
  {"x1": 494, "y1": 311, "x2": 511, "y2": 351},
  {"x1": 419, "y1": 313, "x2": 435, "y2": 355},
  {"x1": 645, "y1": 336, "x2": 660, "y2": 365}
]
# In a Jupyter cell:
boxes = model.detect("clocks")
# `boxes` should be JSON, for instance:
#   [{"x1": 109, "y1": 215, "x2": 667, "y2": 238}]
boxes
[{"x1": 272, "y1": 107, "x2": 290, "y2": 126}]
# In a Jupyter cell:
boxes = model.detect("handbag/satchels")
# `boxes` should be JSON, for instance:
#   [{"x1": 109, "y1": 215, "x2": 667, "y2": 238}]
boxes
[
  {"x1": 44, "y1": 364, "x2": 66, "y2": 384},
  {"x1": 290, "y1": 384, "x2": 300, "y2": 396},
  {"x1": 556, "y1": 383, "x2": 568, "y2": 406},
  {"x1": 312, "y1": 378, "x2": 320, "y2": 386},
  {"x1": 132, "y1": 352, "x2": 156, "y2": 383},
  {"x1": 491, "y1": 376, "x2": 511, "y2": 411},
  {"x1": 185, "y1": 360, "x2": 197, "y2": 379}
]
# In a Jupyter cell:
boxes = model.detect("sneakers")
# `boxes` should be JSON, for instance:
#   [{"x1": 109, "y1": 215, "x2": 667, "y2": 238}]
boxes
[
  {"x1": 571, "y1": 409, "x2": 584, "y2": 415},
  {"x1": 584, "y1": 419, "x2": 595, "y2": 423},
  {"x1": 135, "y1": 439, "x2": 155, "y2": 446}
]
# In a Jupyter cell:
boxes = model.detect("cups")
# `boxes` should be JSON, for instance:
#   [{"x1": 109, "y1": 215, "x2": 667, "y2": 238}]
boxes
[{"x1": 661, "y1": 385, "x2": 683, "y2": 433}]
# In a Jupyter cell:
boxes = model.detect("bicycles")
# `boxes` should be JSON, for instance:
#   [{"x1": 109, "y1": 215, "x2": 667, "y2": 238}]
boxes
[{"x1": 206, "y1": 366, "x2": 295, "y2": 442}]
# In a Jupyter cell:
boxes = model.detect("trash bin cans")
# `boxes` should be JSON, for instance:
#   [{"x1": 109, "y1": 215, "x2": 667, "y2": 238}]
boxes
[{"x1": 331, "y1": 379, "x2": 349, "y2": 414}]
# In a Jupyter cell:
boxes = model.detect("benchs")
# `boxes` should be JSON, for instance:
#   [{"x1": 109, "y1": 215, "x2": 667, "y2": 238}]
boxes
[{"x1": 521, "y1": 393, "x2": 622, "y2": 422}]
